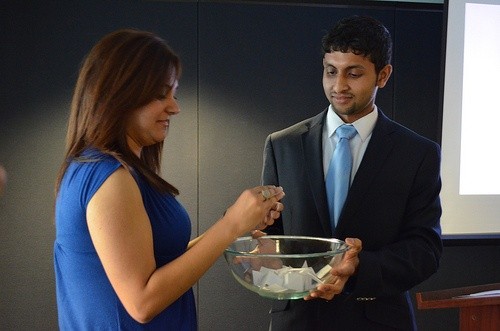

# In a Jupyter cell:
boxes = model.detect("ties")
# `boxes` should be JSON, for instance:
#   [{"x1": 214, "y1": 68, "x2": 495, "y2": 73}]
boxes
[{"x1": 327, "y1": 125, "x2": 356, "y2": 232}]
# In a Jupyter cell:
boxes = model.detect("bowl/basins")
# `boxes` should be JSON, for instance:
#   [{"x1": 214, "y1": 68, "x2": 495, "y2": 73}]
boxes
[{"x1": 221, "y1": 235, "x2": 352, "y2": 301}]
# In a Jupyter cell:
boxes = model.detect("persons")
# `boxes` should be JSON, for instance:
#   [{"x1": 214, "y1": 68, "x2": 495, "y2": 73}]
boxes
[
  {"x1": 232, "y1": 14, "x2": 444, "y2": 331},
  {"x1": 54, "y1": 30, "x2": 285, "y2": 331}
]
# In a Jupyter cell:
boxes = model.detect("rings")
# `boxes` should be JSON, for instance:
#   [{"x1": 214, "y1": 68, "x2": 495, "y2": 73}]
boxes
[{"x1": 261, "y1": 190, "x2": 273, "y2": 198}]
